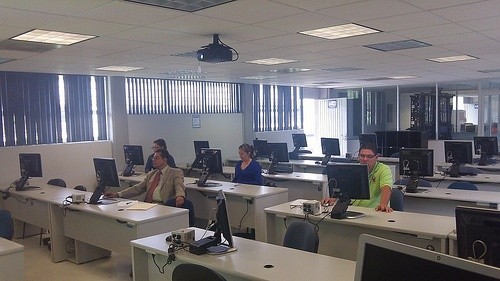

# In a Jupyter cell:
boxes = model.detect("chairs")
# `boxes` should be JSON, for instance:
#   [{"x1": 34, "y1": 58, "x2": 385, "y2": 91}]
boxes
[
  {"x1": 165, "y1": 197, "x2": 196, "y2": 228},
  {"x1": 390, "y1": 188, "x2": 403, "y2": 212},
  {"x1": 283, "y1": 220, "x2": 320, "y2": 253}
]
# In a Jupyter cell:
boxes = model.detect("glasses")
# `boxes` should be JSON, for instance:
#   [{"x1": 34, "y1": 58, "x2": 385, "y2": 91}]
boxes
[
  {"x1": 151, "y1": 146, "x2": 162, "y2": 150},
  {"x1": 358, "y1": 154, "x2": 377, "y2": 159}
]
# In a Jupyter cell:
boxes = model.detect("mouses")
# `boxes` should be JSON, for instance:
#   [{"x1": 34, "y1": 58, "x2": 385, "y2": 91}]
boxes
[
  {"x1": 323, "y1": 203, "x2": 331, "y2": 207},
  {"x1": 102, "y1": 195, "x2": 111, "y2": 199}
]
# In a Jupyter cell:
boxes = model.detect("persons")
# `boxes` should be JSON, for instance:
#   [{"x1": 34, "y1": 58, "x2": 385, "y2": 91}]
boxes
[
  {"x1": 103, "y1": 149, "x2": 185, "y2": 208},
  {"x1": 486, "y1": 123, "x2": 499, "y2": 136},
  {"x1": 144, "y1": 139, "x2": 176, "y2": 174},
  {"x1": 320, "y1": 143, "x2": 394, "y2": 213},
  {"x1": 231, "y1": 143, "x2": 263, "y2": 186}
]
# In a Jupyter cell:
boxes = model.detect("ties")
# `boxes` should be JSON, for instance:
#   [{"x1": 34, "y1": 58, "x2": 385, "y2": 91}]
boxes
[{"x1": 144, "y1": 171, "x2": 162, "y2": 203}]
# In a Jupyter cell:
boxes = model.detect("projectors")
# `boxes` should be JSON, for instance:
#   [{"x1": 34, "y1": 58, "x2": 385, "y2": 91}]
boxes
[{"x1": 195, "y1": 46, "x2": 233, "y2": 63}]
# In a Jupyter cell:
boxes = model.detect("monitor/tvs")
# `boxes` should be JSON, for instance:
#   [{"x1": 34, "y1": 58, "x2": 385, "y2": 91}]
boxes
[
  {"x1": 87, "y1": 157, "x2": 120, "y2": 204},
  {"x1": 253, "y1": 139, "x2": 267, "y2": 160},
  {"x1": 191, "y1": 140, "x2": 209, "y2": 169},
  {"x1": 353, "y1": 233, "x2": 500, "y2": 281},
  {"x1": 292, "y1": 133, "x2": 307, "y2": 154},
  {"x1": 454, "y1": 206, "x2": 500, "y2": 270},
  {"x1": 266, "y1": 142, "x2": 289, "y2": 173},
  {"x1": 208, "y1": 189, "x2": 234, "y2": 253},
  {"x1": 195, "y1": 148, "x2": 222, "y2": 187},
  {"x1": 358, "y1": 133, "x2": 377, "y2": 151},
  {"x1": 320, "y1": 137, "x2": 339, "y2": 165},
  {"x1": 120, "y1": 144, "x2": 144, "y2": 175},
  {"x1": 15, "y1": 153, "x2": 42, "y2": 191},
  {"x1": 397, "y1": 135, "x2": 498, "y2": 193},
  {"x1": 325, "y1": 163, "x2": 371, "y2": 219}
]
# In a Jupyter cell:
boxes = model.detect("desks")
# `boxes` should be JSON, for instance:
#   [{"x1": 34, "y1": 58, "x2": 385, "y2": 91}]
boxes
[{"x1": 1, "y1": 157, "x2": 500, "y2": 281}]
